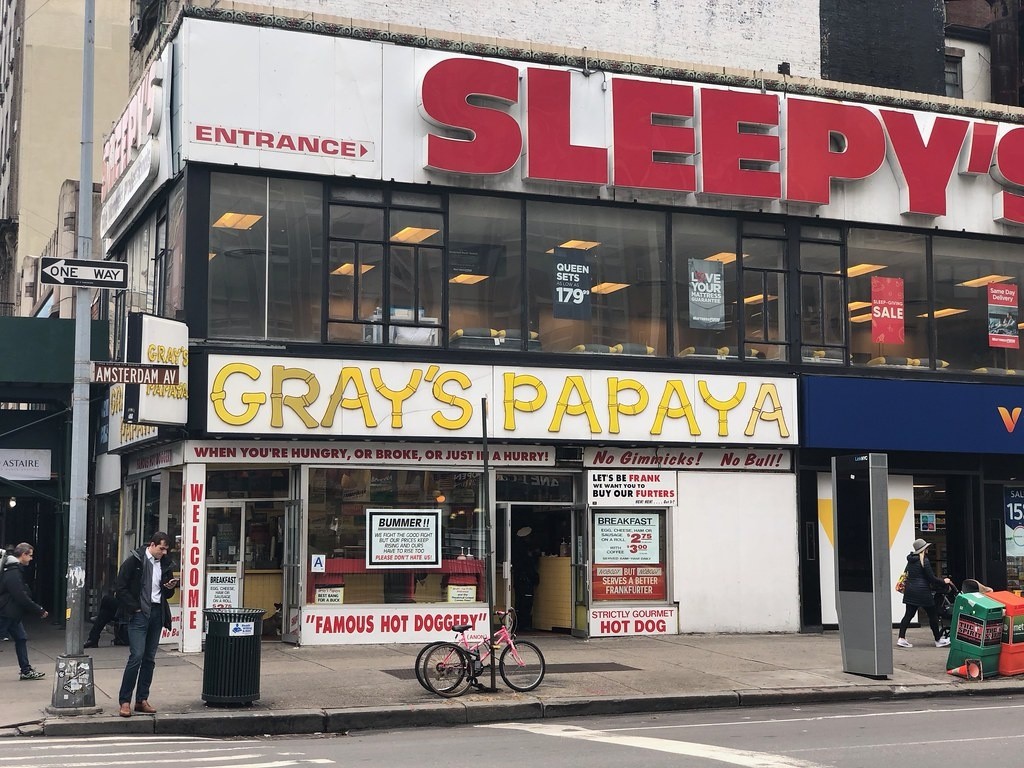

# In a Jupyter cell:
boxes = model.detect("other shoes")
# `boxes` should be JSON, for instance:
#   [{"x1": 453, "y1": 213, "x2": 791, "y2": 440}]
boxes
[
  {"x1": 522, "y1": 626, "x2": 537, "y2": 632},
  {"x1": 134, "y1": 700, "x2": 157, "y2": 713},
  {"x1": 83, "y1": 638, "x2": 98, "y2": 648},
  {"x1": 119, "y1": 702, "x2": 132, "y2": 717},
  {"x1": 19, "y1": 668, "x2": 46, "y2": 680}
]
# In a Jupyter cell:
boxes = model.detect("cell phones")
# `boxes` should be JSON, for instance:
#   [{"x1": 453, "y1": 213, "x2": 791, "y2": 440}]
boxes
[{"x1": 169, "y1": 579, "x2": 176, "y2": 584}]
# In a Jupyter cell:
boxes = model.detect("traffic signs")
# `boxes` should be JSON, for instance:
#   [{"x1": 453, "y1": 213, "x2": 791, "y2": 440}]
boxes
[{"x1": 39, "y1": 256, "x2": 128, "y2": 290}]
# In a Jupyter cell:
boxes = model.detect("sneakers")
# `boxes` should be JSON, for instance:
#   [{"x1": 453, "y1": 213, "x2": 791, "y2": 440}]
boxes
[
  {"x1": 934, "y1": 638, "x2": 950, "y2": 647},
  {"x1": 896, "y1": 638, "x2": 913, "y2": 647}
]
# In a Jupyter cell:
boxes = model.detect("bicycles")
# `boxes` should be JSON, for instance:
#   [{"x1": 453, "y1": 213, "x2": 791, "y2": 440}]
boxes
[{"x1": 415, "y1": 606, "x2": 545, "y2": 698}]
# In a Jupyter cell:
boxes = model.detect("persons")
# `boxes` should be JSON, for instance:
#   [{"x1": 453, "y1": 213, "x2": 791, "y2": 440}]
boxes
[
  {"x1": 897, "y1": 538, "x2": 952, "y2": 650},
  {"x1": 0, "y1": 541, "x2": 49, "y2": 681},
  {"x1": 514, "y1": 537, "x2": 541, "y2": 633},
  {"x1": 83, "y1": 531, "x2": 181, "y2": 717}
]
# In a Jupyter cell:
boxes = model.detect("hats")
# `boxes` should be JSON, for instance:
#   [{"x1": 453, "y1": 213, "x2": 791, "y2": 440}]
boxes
[
  {"x1": 517, "y1": 526, "x2": 533, "y2": 537},
  {"x1": 912, "y1": 539, "x2": 932, "y2": 554}
]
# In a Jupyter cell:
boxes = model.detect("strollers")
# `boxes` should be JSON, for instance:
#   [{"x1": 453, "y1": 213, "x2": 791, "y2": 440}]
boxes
[{"x1": 933, "y1": 579, "x2": 993, "y2": 637}]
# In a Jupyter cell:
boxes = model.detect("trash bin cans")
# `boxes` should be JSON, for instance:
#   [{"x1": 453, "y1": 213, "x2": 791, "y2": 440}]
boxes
[{"x1": 202, "y1": 608, "x2": 267, "y2": 708}]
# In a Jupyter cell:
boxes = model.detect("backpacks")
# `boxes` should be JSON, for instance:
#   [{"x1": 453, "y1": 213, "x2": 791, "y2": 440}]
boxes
[{"x1": 0, "y1": 549, "x2": 24, "y2": 576}]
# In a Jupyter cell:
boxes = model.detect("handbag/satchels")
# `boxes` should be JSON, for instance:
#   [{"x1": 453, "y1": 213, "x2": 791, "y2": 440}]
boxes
[{"x1": 895, "y1": 571, "x2": 909, "y2": 594}]
